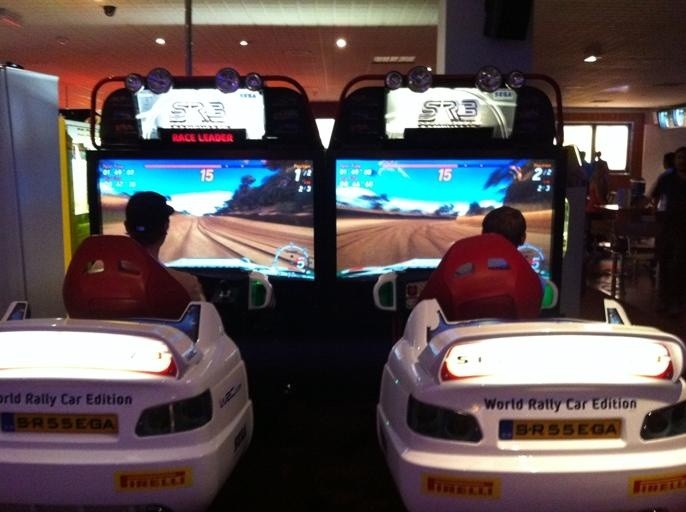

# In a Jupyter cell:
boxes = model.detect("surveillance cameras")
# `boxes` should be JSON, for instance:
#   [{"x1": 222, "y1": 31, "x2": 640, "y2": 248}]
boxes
[{"x1": 102, "y1": 2, "x2": 117, "y2": 17}]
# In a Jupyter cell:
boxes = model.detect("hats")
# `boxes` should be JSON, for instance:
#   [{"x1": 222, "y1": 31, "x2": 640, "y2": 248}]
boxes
[{"x1": 124, "y1": 192, "x2": 174, "y2": 222}]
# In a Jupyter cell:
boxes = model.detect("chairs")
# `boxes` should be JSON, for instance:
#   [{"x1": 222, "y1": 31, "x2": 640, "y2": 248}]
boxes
[
  {"x1": 415, "y1": 234, "x2": 544, "y2": 320},
  {"x1": 63, "y1": 234, "x2": 191, "y2": 321}
]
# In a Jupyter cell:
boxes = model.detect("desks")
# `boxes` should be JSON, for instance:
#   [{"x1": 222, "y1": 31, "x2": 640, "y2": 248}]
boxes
[{"x1": 595, "y1": 202, "x2": 664, "y2": 299}]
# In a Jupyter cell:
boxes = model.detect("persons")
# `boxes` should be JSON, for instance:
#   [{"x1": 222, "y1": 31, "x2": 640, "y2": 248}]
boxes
[
  {"x1": 124, "y1": 190, "x2": 207, "y2": 305},
  {"x1": 580, "y1": 151, "x2": 590, "y2": 170},
  {"x1": 646, "y1": 151, "x2": 675, "y2": 294},
  {"x1": 594, "y1": 150, "x2": 610, "y2": 203},
  {"x1": 649, "y1": 148, "x2": 686, "y2": 319},
  {"x1": 481, "y1": 206, "x2": 558, "y2": 309}
]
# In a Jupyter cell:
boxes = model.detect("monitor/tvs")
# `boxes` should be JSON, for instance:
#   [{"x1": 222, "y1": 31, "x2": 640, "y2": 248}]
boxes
[
  {"x1": 657, "y1": 106, "x2": 686, "y2": 129},
  {"x1": 335, "y1": 158, "x2": 556, "y2": 279},
  {"x1": 97, "y1": 158, "x2": 315, "y2": 284}
]
